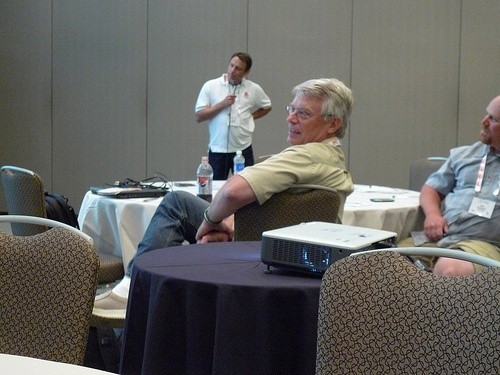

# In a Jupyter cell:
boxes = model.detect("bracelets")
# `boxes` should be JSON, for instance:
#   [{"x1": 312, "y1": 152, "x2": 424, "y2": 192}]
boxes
[{"x1": 203, "y1": 207, "x2": 222, "y2": 226}]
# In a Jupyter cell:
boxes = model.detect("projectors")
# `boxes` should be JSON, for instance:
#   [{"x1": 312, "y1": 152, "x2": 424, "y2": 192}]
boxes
[{"x1": 261, "y1": 220, "x2": 398, "y2": 276}]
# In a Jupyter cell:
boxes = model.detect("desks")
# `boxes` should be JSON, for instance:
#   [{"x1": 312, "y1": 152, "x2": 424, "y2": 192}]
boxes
[
  {"x1": 76, "y1": 176, "x2": 228, "y2": 272},
  {"x1": 118, "y1": 240, "x2": 321, "y2": 375},
  {"x1": 343, "y1": 182, "x2": 425, "y2": 245}
]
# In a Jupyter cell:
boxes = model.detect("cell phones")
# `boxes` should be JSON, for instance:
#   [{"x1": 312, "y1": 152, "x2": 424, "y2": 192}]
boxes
[{"x1": 371, "y1": 198, "x2": 394, "y2": 202}]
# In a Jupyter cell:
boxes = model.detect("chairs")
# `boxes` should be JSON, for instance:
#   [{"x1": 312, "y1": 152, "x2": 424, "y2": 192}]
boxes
[
  {"x1": 314, "y1": 246, "x2": 500, "y2": 375},
  {"x1": 0, "y1": 212, "x2": 126, "y2": 375},
  {"x1": 0, "y1": 164, "x2": 124, "y2": 283}
]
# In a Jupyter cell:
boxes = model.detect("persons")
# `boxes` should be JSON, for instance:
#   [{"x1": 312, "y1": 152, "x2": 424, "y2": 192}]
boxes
[
  {"x1": 194, "y1": 52, "x2": 273, "y2": 181},
  {"x1": 398, "y1": 95, "x2": 500, "y2": 275},
  {"x1": 90, "y1": 78, "x2": 354, "y2": 318}
]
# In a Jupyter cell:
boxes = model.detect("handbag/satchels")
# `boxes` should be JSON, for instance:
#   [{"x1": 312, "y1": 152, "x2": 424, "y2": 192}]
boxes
[{"x1": 44, "y1": 190, "x2": 80, "y2": 232}]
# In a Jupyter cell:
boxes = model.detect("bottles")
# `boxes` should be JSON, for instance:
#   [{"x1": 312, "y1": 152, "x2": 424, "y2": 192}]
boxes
[
  {"x1": 232, "y1": 150, "x2": 245, "y2": 176},
  {"x1": 197, "y1": 156, "x2": 213, "y2": 194}
]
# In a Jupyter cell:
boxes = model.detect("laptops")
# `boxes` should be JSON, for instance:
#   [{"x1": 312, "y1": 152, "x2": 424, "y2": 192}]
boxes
[{"x1": 91, "y1": 187, "x2": 170, "y2": 199}]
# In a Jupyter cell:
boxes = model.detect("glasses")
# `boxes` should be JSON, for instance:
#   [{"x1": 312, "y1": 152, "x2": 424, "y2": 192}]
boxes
[{"x1": 286, "y1": 104, "x2": 312, "y2": 119}]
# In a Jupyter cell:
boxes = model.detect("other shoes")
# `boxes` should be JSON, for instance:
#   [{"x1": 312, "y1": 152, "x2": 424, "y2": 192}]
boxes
[{"x1": 92, "y1": 286, "x2": 128, "y2": 319}]
